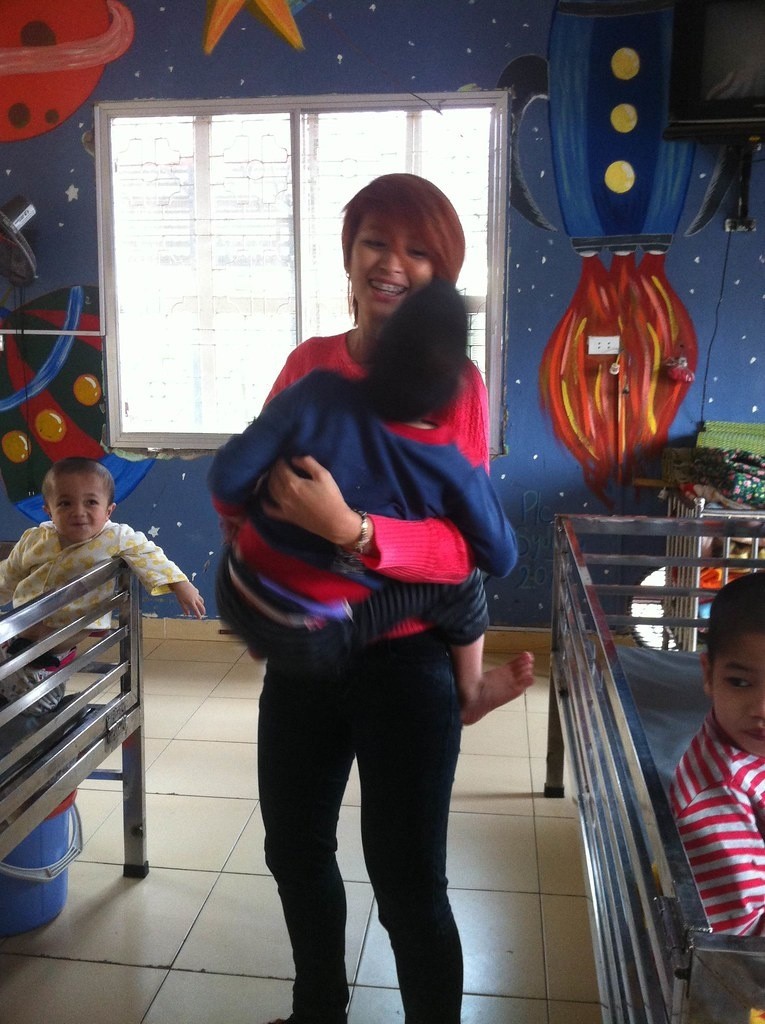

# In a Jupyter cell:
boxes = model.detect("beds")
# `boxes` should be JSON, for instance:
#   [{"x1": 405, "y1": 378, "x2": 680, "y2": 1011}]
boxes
[
  {"x1": 544, "y1": 512, "x2": 765, "y2": 1024},
  {"x1": 0, "y1": 552, "x2": 153, "y2": 881},
  {"x1": 660, "y1": 480, "x2": 765, "y2": 654}
]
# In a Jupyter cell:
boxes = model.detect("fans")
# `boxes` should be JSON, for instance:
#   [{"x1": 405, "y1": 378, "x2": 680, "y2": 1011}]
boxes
[{"x1": 0, "y1": 196, "x2": 40, "y2": 288}]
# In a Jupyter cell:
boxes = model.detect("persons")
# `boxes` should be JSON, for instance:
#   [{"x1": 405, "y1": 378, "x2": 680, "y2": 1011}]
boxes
[
  {"x1": 668, "y1": 571, "x2": 765, "y2": 937},
  {"x1": 212, "y1": 174, "x2": 489, "y2": 1024},
  {"x1": 210, "y1": 281, "x2": 534, "y2": 725},
  {"x1": 0, "y1": 459, "x2": 205, "y2": 711}
]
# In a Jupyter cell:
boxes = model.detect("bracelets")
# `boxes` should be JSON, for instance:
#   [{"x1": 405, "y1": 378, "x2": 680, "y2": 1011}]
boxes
[{"x1": 340, "y1": 511, "x2": 370, "y2": 558}]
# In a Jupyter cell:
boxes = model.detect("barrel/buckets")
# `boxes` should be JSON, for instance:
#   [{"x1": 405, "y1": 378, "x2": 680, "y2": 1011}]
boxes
[{"x1": 0, "y1": 788, "x2": 84, "y2": 937}]
